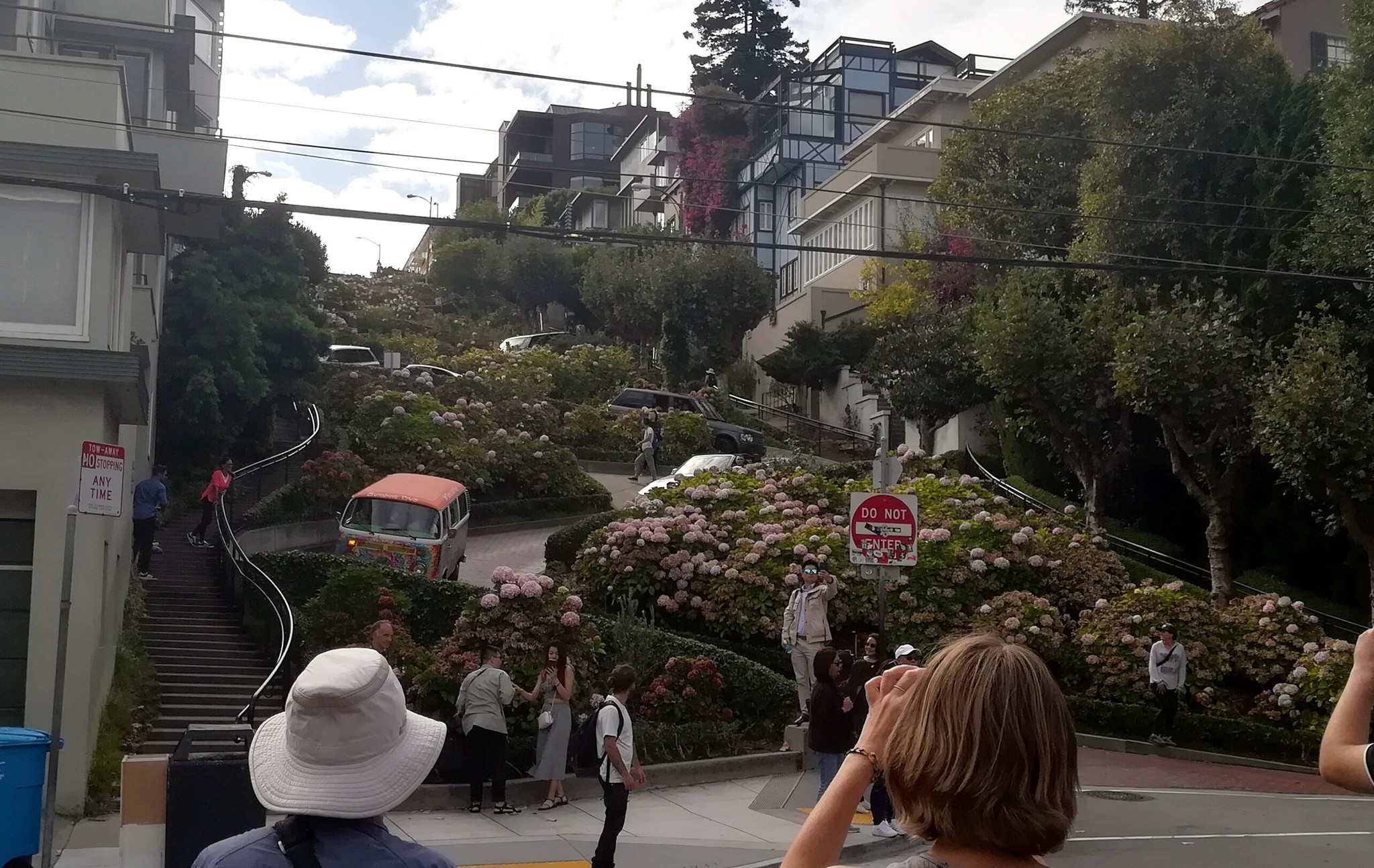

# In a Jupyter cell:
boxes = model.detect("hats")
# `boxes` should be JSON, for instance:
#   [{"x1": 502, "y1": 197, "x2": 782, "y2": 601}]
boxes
[
  {"x1": 247, "y1": 647, "x2": 447, "y2": 819},
  {"x1": 804, "y1": 559, "x2": 819, "y2": 568},
  {"x1": 895, "y1": 644, "x2": 922, "y2": 660},
  {"x1": 705, "y1": 368, "x2": 715, "y2": 374}
]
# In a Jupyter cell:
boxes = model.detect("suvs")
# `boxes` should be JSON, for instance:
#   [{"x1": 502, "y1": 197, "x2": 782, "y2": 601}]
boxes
[
  {"x1": 317, "y1": 345, "x2": 380, "y2": 367},
  {"x1": 499, "y1": 332, "x2": 569, "y2": 350},
  {"x1": 608, "y1": 388, "x2": 767, "y2": 463}
]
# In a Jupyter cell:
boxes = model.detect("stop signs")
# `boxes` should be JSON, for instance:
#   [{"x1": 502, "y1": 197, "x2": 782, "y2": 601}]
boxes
[{"x1": 849, "y1": 492, "x2": 918, "y2": 565}]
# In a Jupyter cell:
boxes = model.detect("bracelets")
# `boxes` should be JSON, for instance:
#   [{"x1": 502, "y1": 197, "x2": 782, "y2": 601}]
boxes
[
  {"x1": 555, "y1": 682, "x2": 560, "y2": 687},
  {"x1": 845, "y1": 747, "x2": 879, "y2": 784}
]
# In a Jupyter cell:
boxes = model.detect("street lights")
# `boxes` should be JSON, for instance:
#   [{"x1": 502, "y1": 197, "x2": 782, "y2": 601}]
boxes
[
  {"x1": 356, "y1": 237, "x2": 380, "y2": 261},
  {"x1": 631, "y1": 182, "x2": 684, "y2": 252},
  {"x1": 232, "y1": 165, "x2": 272, "y2": 200},
  {"x1": 433, "y1": 202, "x2": 438, "y2": 218},
  {"x1": 407, "y1": 195, "x2": 432, "y2": 284}
]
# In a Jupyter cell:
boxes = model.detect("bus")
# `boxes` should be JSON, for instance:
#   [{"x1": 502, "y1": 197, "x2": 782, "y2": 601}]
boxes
[{"x1": 332, "y1": 473, "x2": 471, "y2": 580}]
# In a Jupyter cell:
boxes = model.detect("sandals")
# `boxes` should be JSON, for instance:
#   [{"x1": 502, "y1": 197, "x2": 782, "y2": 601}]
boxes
[
  {"x1": 538, "y1": 798, "x2": 557, "y2": 810},
  {"x1": 493, "y1": 801, "x2": 522, "y2": 814},
  {"x1": 469, "y1": 802, "x2": 482, "y2": 812},
  {"x1": 554, "y1": 794, "x2": 568, "y2": 805}
]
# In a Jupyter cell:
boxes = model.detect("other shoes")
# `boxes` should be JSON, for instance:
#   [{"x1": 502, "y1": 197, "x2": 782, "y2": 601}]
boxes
[
  {"x1": 628, "y1": 477, "x2": 638, "y2": 482},
  {"x1": 646, "y1": 480, "x2": 654, "y2": 485},
  {"x1": 794, "y1": 714, "x2": 810, "y2": 725}
]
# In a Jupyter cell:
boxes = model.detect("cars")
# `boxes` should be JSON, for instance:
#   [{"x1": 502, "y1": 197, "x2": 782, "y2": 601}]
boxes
[
  {"x1": 638, "y1": 454, "x2": 753, "y2": 494},
  {"x1": 405, "y1": 364, "x2": 463, "y2": 379}
]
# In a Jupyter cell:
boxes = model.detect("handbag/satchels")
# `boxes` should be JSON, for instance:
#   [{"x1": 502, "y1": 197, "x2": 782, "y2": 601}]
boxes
[{"x1": 537, "y1": 710, "x2": 554, "y2": 730}]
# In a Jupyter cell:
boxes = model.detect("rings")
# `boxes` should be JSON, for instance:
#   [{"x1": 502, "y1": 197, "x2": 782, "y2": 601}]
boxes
[{"x1": 894, "y1": 685, "x2": 906, "y2": 692}]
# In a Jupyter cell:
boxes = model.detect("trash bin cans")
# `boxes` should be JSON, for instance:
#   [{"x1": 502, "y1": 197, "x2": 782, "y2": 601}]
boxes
[
  {"x1": 165, "y1": 723, "x2": 267, "y2": 868},
  {"x1": 0, "y1": 726, "x2": 64, "y2": 868},
  {"x1": 119, "y1": 754, "x2": 170, "y2": 868}
]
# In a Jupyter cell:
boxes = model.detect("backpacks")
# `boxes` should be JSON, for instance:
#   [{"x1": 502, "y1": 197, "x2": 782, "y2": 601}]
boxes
[
  {"x1": 652, "y1": 435, "x2": 658, "y2": 449},
  {"x1": 568, "y1": 700, "x2": 623, "y2": 778},
  {"x1": 708, "y1": 374, "x2": 717, "y2": 387}
]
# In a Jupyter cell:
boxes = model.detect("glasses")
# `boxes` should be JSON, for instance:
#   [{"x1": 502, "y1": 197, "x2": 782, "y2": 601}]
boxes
[
  {"x1": 494, "y1": 656, "x2": 502, "y2": 659},
  {"x1": 164, "y1": 474, "x2": 168, "y2": 477},
  {"x1": 866, "y1": 642, "x2": 877, "y2": 648},
  {"x1": 831, "y1": 660, "x2": 842, "y2": 666},
  {"x1": 906, "y1": 656, "x2": 919, "y2": 661},
  {"x1": 804, "y1": 568, "x2": 818, "y2": 574}
]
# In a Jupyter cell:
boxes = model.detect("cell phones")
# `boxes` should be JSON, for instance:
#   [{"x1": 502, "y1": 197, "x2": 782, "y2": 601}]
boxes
[{"x1": 548, "y1": 660, "x2": 556, "y2": 673}]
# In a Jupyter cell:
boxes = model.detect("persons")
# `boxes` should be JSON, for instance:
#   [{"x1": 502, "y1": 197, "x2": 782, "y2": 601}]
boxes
[
  {"x1": 1319, "y1": 626, "x2": 1374, "y2": 794},
  {"x1": 354, "y1": 503, "x2": 438, "y2": 537},
  {"x1": 191, "y1": 647, "x2": 456, "y2": 868},
  {"x1": 1149, "y1": 623, "x2": 1187, "y2": 746},
  {"x1": 735, "y1": 454, "x2": 746, "y2": 467},
  {"x1": 187, "y1": 457, "x2": 235, "y2": 547},
  {"x1": 627, "y1": 418, "x2": 658, "y2": 484},
  {"x1": 705, "y1": 369, "x2": 717, "y2": 387},
  {"x1": 370, "y1": 619, "x2": 646, "y2": 868},
  {"x1": 132, "y1": 464, "x2": 169, "y2": 580},
  {"x1": 781, "y1": 560, "x2": 1081, "y2": 868}
]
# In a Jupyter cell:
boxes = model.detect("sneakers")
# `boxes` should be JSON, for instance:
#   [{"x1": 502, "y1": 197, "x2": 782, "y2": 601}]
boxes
[
  {"x1": 860, "y1": 799, "x2": 871, "y2": 810},
  {"x1": 1148, "y1": 733, "x2": 1177, "y2": 747},
  {"x1": 197, "y1": 540, "x2": 215, "y2": 548},
  {"x1": 847, "y1": 824, "x2": 860, "y2": 833},
  {"x1": 872, "y1": 818, "x2": 898, "y2": 837},
  {"x1": 856, "y1": 802, "x2": 869, "y2": 813},
  {"x1": 139, "y1": 572, "x2": 158, "y2": 580},
  {"x1": 187, "y1": 532, "x2": 197, "y2": 547},
  {"x1": 888, "y1": 818, "x2": 907, "y2": 835}
]
